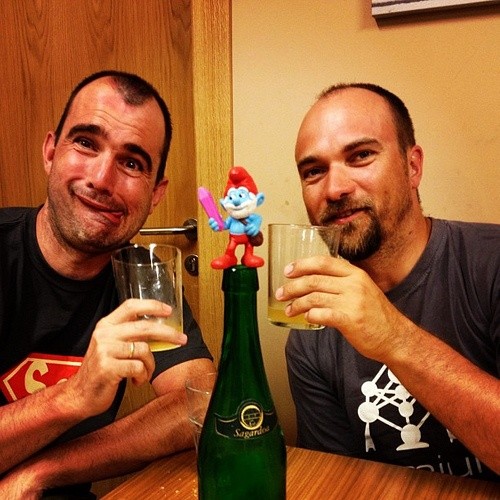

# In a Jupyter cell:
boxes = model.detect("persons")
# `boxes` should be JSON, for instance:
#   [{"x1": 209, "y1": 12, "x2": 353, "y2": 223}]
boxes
[
  {"x1": 0, "y1": 69, "x2": 217, "y2": 500},
  {"x1": 275, "y1": 84, "x2": 499, "y2": 481}
]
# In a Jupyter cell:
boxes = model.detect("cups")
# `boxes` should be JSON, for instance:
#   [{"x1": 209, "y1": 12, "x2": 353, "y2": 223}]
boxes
[
  {"x1": 112, "y1": 243, "x2": 183, "y2": 352},
  {"x1": 185, "y1": 372, "x2": 217, "y2": 451},
  {"x1": 268, "y1": 223, "x2": 342, "y2": 331}
]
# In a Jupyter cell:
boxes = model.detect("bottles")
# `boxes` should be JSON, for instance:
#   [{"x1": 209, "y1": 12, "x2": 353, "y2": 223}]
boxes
[{"x1": 195, "y1": 265, "x2": 287, "y2": 500}]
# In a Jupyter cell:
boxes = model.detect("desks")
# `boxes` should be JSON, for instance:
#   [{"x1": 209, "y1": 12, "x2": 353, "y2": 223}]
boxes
[{"x1": 100, "y1": 442, "x2": 500, "y2": 500}]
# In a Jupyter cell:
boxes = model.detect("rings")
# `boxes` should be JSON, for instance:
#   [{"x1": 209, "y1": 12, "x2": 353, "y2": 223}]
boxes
[{"x1": 129, "y1": 341, "x2": 135, "y2": 358}]
